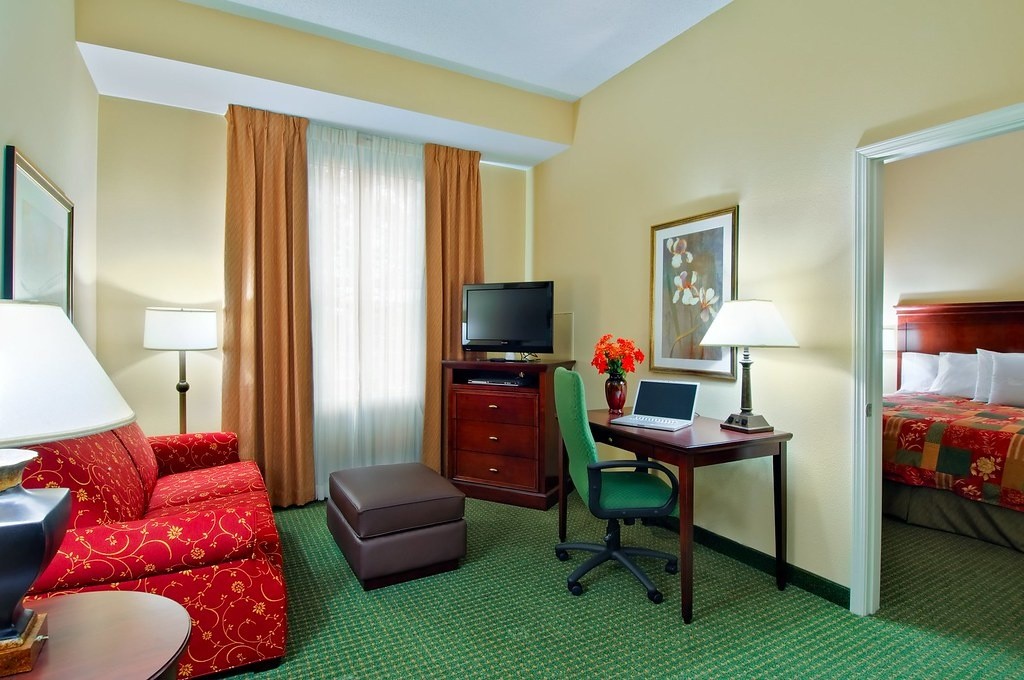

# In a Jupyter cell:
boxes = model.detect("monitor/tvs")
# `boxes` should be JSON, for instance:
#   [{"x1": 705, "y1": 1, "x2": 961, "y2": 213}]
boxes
[{"x1": 462, "y1": 281, "x2": 554, "y2": 362}]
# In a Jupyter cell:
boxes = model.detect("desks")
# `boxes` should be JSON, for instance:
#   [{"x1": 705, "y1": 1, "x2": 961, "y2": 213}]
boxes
[{"x1": 550, "y1": 405, "x2": 796, "y2": 627}]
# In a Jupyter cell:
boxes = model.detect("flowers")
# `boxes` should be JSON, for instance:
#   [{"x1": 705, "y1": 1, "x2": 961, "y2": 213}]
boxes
[{"x1": 590, "y1": 334, "x2": 647, "y2": 376}]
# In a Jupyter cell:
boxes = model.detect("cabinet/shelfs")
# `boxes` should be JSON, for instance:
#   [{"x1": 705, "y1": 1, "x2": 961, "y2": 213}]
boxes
[{"x1": 435, "y1": 358, "x2": 578, "y2": 512}]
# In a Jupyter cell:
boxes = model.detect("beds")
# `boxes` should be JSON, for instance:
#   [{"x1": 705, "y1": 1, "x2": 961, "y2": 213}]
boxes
[{"x1": 881, "y1": 297, "x2": 1024, "y2": 557}]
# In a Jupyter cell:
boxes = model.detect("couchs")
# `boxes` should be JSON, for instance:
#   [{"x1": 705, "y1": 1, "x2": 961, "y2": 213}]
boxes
[{"x1": 19, "y1": 417, "x2": 291, "y2": 680}]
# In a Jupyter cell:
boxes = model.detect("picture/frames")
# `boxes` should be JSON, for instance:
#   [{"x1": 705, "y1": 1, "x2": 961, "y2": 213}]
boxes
[
  {"x1": 4, "y1": 143, "x2": 76, "y2": 325},
  {"x1": 644, "y1": 203, "x2": 746, "y2": 384}
]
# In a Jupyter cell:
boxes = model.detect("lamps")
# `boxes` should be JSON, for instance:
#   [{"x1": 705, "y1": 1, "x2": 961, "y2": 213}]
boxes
[
  {"x1": 698, "y1": 296, "x2": 806, "y2": 431},
  {"x1": 140, "y1": 305, "x2": 221, "y2": 436},
  {"x1": 0, "y1": 298, "x2": 138, "y2": 678}
]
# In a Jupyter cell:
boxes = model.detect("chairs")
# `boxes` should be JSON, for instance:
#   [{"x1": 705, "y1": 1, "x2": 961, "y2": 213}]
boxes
[{"x1": 549, "y1": 366, "x2": 683, "y2": 607}]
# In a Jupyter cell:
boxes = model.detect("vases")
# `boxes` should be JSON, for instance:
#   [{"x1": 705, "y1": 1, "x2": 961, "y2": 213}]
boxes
[{"x1": 603, "y1": 372, "x2": 628, "y2": 416}]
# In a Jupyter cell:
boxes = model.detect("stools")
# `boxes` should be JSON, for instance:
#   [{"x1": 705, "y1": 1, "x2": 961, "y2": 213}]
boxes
[{"x1": 324, "y1": 460, "x2": 471, "y2": 593}]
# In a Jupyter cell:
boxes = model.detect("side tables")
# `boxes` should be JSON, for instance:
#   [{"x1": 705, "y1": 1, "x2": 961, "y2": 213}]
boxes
[{"x1": 0, "y1": 587, "x2": 194, "y2": 680}]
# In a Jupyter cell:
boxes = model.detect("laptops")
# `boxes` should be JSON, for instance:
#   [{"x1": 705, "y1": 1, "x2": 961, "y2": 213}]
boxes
[{"x1": 610, "y1": 380, "x2": 700, "y2": 432}]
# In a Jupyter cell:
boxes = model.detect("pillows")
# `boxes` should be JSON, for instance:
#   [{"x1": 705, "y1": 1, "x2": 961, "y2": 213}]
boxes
[
  {"x1": 927, "y1": 351, "x2": 978, "y2": 398},
  {"x1": 969, "y1": 347, "x2": 994, "y2": 403},
  {"x1": 894, "y1": 351, "x2": 938, "y2": 395},
  {"x1": 986, "y1": 351, "x2": 1024, "y2": 408}
]
